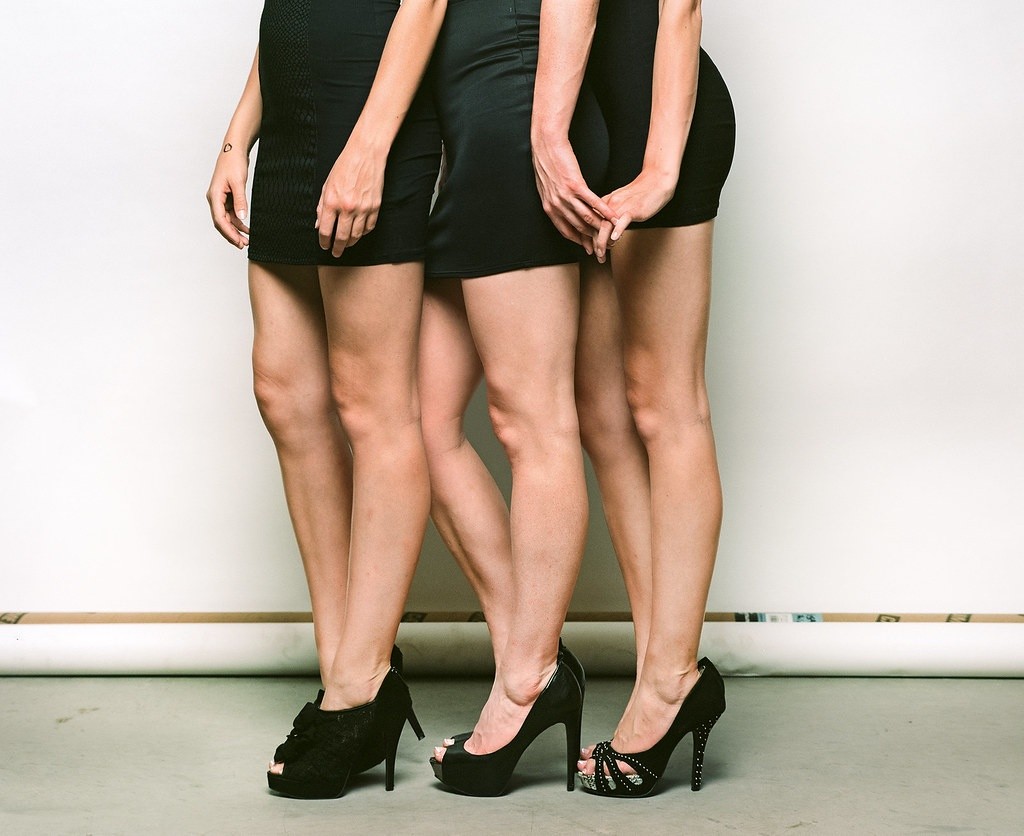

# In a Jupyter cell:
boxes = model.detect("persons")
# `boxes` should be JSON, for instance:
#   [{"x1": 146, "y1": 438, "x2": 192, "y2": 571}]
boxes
[
  {"x1": 209, "y1": 0, "x2": 448, "y2": 799},
  {"x1": 420, "y1": 1, "x2": 619, "y2": 798},
  {"x1": 578, "y1": 1, "x2": 737, "y2": 799}
]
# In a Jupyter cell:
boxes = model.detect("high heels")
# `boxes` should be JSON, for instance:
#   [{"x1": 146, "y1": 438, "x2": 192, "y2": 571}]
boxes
[
  {"x1": 429, "y1": 649, "x2": 584, "y2": 798},
  {"x1": 443, "y1": 635, "x2": 586, "y2": 773},
  {"x1": 267, "y1": 666, "x2": 412, "y2": 799},
  {"x1": 274, "y1": 644, "x2": 426, "y2": 776},
  {"x1": 577, "y1": 655, "x2": 726, "y2": 799}
]
